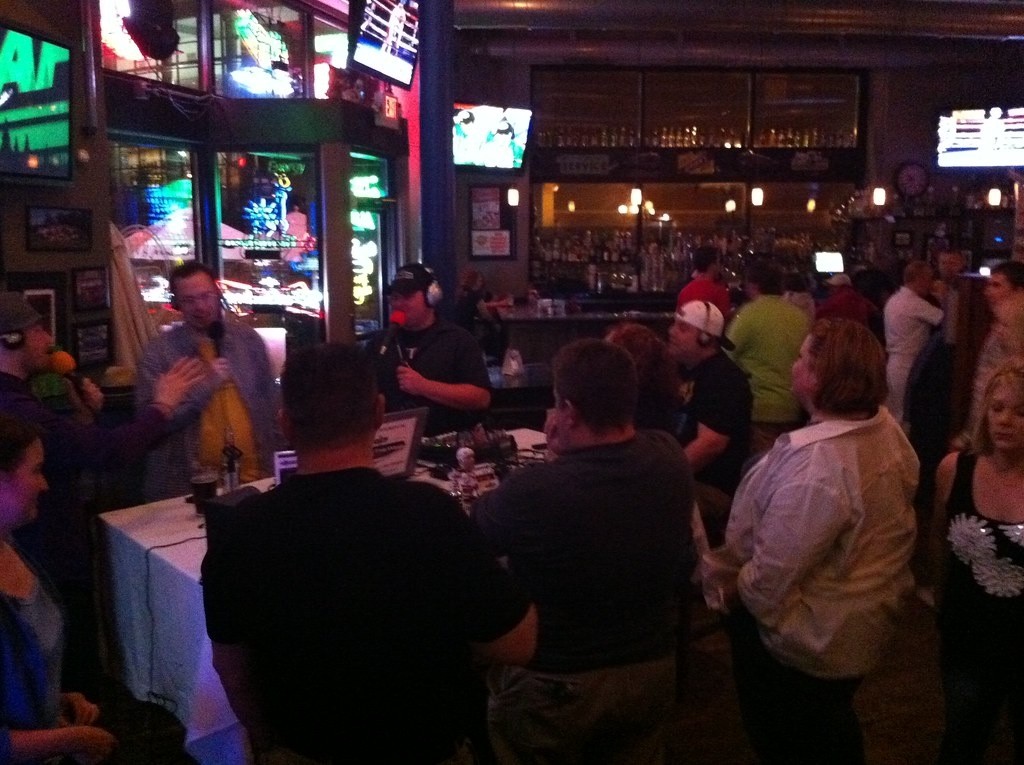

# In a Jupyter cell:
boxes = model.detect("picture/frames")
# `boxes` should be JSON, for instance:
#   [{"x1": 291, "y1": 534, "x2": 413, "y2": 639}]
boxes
[{"x1": 24, "y1": 204, "x2": 93, "y2": 252}]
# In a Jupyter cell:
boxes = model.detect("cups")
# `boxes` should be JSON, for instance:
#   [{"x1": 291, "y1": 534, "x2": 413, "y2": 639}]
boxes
[{"x1": 191, "y1": 461, "x2": 219, "y2": 516}]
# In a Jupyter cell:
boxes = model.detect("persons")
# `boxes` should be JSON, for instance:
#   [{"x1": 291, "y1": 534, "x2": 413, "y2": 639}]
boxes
[
  {"x1": 0, "y1": 289, "x2": 207, "y2": 694},
  {"x1": 200, "y1": 338, "x2": 536, "y2": 765},
  {"x1": 447, "y1": 448, "x2": 485, "y2": 517},
  {"x1": 782, "y1": 271, "x2": 815, "y2": 320},
  {"x1": 0, "y1": 417, "x2": 201, "y2": 765},
  {"x1": 884, "y1": 245, "x2": 1024, "y2": 765},
  {"x1": 721, "y1": 262, "x2": 812, "y2": 461},
  {"x1": 453, "y1": 263, "x2": 514, "y2": 323},
  {"x1": 601, "y1": 323, "x2": 735, "y2": 673},
  {"x1": 670, "y1": 301, "x2": 753, "y2": 552},
  {"x1": 678, "y1": 244, "x2": 735, "y2": 323},
  {"x1": 368, "y1": 260, "x2": 493, "y2": 438},
  {"x1": 472, "y1": 339, "x2": 698, "y2": 765},
  {"x1": 691, "y1": 316, "x2": 920, "y2": 765},
  {"x1": 133, "y1": 260, "x2": 290, "y2": 503}
]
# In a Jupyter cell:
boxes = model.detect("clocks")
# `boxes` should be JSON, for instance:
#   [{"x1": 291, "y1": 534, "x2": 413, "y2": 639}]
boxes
[{"x1": 893, "y1": 160, "x2": 931, "y2": 198}]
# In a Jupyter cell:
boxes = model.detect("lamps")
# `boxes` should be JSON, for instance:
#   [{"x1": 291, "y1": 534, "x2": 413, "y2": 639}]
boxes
[{"x1": 123, "y1": 14, "x2": 178, "y2": 60}]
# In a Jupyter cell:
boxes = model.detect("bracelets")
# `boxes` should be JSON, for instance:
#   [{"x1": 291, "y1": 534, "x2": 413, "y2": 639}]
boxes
[
  {"x1": 146, "y1": 397, "x2": 174, "y2": 411},
  {"x1": 692, "y1": 620, "x2": 721, "y2": 639}
]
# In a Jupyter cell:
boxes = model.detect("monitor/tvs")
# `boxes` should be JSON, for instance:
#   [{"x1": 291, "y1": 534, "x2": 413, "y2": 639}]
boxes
[
  {"x1": 346, "y1": 0, "x2": 422, "y2": 91},
  {"x1": 813, "y1": 251, "x2": 845, "y2": 274},
  {"x1": 450, "y1": 99, "x2": 537, "y2": 178},
  {"x1": 934, "y1": 105, "x2": 1024, "y2": 173}
]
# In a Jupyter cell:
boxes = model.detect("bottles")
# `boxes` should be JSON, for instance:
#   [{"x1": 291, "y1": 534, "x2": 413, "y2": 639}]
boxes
[
  {"x1": 545, "y1": 230, "x2": 823, "y2": 263},
  {"x1": 538, "y1": 126, "x2": 857, "y2": 147}
]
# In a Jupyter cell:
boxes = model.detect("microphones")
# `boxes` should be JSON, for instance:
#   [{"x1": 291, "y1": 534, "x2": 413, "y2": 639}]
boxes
[
  {"x1": 49, "y1": 351, "x2": 86, "y2": 399},
  {"x1": 207, "y1": 320, "x2": 225, "y2": 359},
  {"x1": 380, "y1": 311, "x2": 408, "y2": 355}
]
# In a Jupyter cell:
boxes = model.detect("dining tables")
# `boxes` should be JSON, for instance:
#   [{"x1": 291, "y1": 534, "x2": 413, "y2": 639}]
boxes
[{"x1": 97, "y1": 426, "x2": 548, "y2": 765}]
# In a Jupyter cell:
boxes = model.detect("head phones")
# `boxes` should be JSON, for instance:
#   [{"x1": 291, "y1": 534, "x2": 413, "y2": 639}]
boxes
[
  {"x1": 0, "y1": 329, "x2": 24, "y2": 350},
  {"x1": 424, "y1": 267, "x2": 443, "y2": 308},
  {"x1": 697, "y1": 301, "x2": 712, "y2": 348}
]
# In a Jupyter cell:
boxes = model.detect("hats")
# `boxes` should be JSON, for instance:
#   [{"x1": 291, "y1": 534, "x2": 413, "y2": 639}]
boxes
[
  {"x1": 677, "y1": 299, "x2": 736, "y2": 351},
  {"x1": 0, "y1": 292, "x2": 43, "y2": 334},
  {"x1": 383, "y1": 263, "x2": 432, "y2": 295}
]
